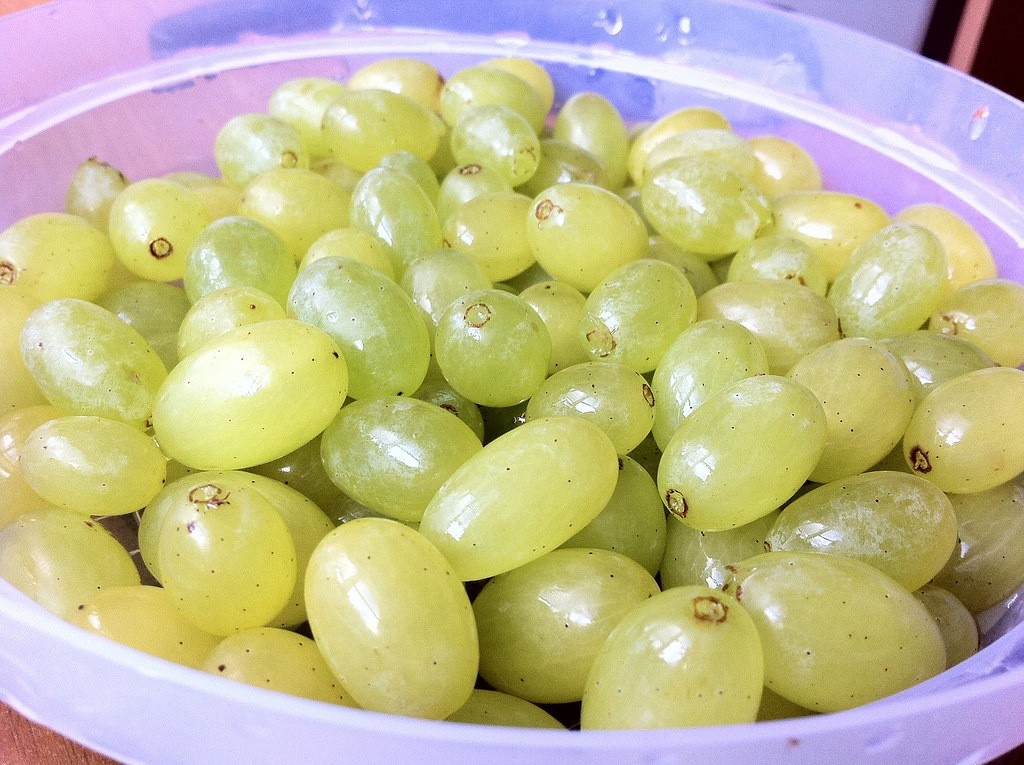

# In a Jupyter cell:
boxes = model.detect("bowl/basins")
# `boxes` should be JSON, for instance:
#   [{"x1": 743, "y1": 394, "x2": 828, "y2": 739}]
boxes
[{"x1": 1, "y1": 0, "x2": 1024, "y2": 765}]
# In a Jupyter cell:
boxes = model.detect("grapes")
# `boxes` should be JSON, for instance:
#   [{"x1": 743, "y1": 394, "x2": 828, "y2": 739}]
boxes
[{"x1": 0, "y1": 58, "x2": 1024, "y2": 732}]
